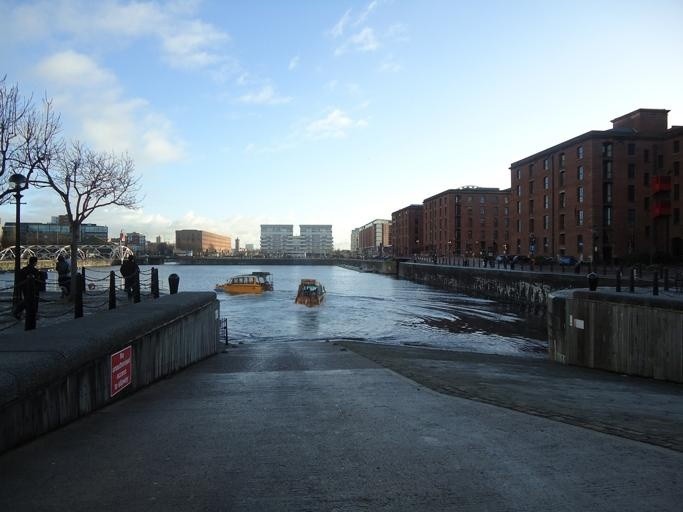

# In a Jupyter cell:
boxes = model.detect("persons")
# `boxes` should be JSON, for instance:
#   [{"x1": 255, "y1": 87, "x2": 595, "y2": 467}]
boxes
[
  {"x1": 13, "y1": 257, "x2": 42, "y2": 320},
  {"x1": 56, "y1": 255, "x2": 69, "y2": 298},
  {"x1": 120, "y1": 255, "x2": 139, "y2": 292}
]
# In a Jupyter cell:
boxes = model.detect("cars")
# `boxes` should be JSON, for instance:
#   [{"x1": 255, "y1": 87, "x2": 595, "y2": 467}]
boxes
[{"x1": 498, "y1": 255, "x2": 578, "y2": 265}]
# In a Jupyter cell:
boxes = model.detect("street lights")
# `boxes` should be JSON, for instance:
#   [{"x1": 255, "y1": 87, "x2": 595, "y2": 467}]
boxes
[{"x1": 8, "y1": 174, "x2": 26, "y2": 314}]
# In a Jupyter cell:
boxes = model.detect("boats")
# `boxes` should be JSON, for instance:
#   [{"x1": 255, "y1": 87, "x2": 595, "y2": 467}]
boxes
[
  {"x1": 295, "y1": 278, "x2": 326, "y2": 309},
  {"x1": 215, "y1": 272, "x2": 274, "y2": 294}
]
